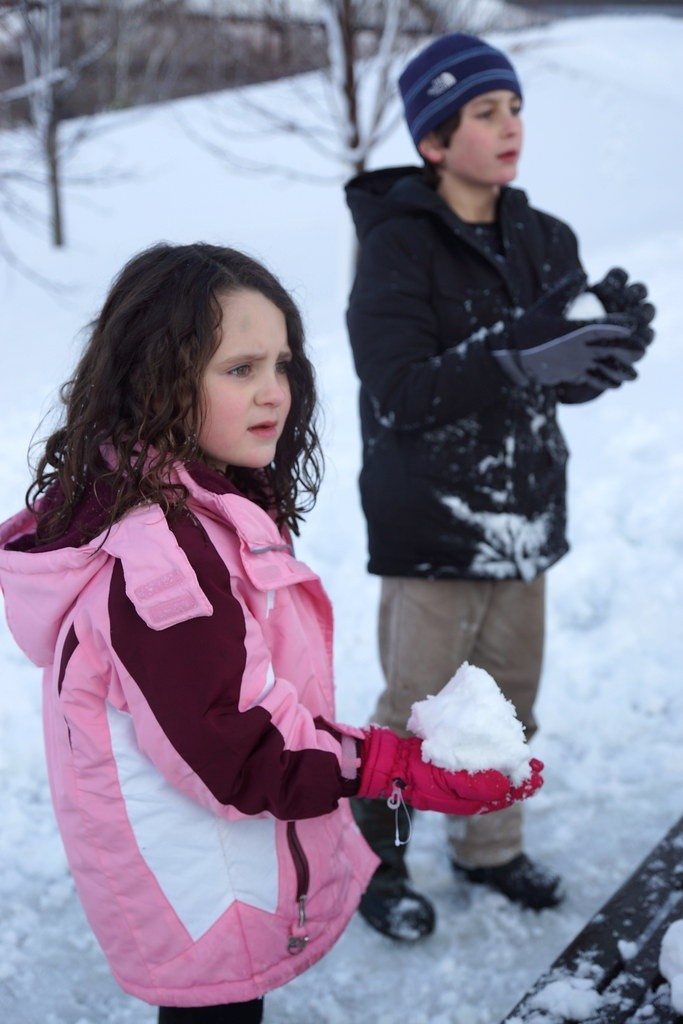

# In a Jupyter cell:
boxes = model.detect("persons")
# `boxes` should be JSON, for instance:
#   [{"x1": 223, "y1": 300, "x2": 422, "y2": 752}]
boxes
[
  {"x1": 1, "y1": 241, "x2": 545, "y2": 1024},
  {"x1": 344, "y1": 34, "x2": 657, "y2": 941}
]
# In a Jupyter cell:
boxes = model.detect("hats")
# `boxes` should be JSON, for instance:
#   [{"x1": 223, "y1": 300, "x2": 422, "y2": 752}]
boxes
[{"x1": 397, "y1": 33, "x2": 523, "y2": 151}]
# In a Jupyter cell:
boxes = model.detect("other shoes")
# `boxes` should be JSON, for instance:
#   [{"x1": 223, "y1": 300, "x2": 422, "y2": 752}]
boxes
[
  {"x1": 488, "y1": 857, "x2": 567, "y2": 911},
  {"x1": 357, "y1": 886, "x2": 436, "y2": 938}
]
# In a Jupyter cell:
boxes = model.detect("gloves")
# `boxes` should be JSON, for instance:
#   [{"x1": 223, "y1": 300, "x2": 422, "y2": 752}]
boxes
[
  {"x1": 549, "y1": 268, "x2": 657, "y2": 406},
  {"x1": 354, "y1": 723, "x2": 546, "y2": 816},
  {"x1": 484, "y1": 268, "x2": 646, "y2": 394}
]
